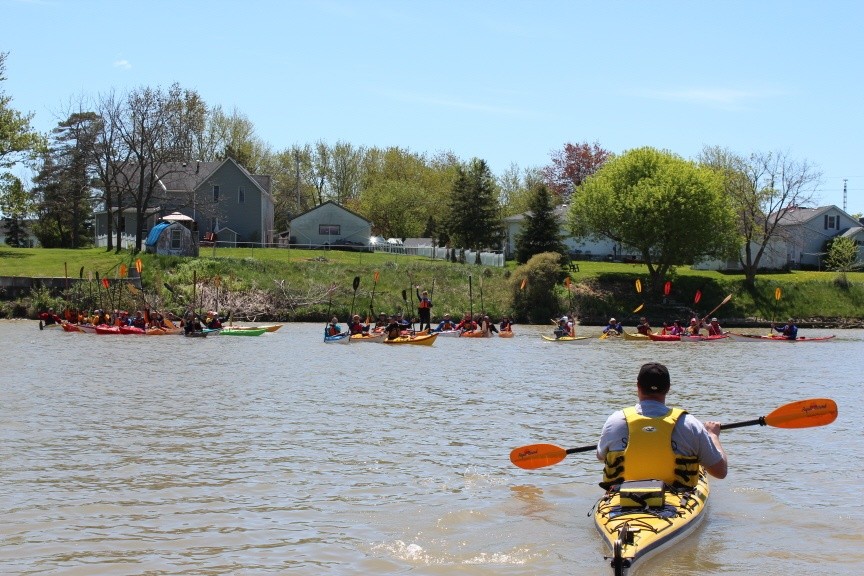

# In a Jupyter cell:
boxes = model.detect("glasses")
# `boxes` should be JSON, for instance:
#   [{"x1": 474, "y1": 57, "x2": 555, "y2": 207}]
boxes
[
  {"x1": 504, "y1": 318, "x2": 508, "y2": 319},
  {"x1": 641, "y1": 320, "x2": 644, "y2": 321}
]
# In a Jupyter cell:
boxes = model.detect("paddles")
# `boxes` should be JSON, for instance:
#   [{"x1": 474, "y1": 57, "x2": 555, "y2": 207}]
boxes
[
  {"x1": 125, "y1": 282, "x2": 176, "y2": 329},
  {"x1": 509, "y1": 397, "x2": 839, "y2": 470},
  {"x1": 769, "y1": 287, "x2": 781, "y2": 336},
  {"x1": 550, "y1": 318, "x2": 574, "y2": 337},
  {"x1": 599, "y1": 302, "x2": 645, "y2": 340},
  {"x1": 506, "y1": 276, "x2": 527, "y2": 331},
  {"x1": 682, "y1": 290, "x2": 702, "y2": 334},
  {"x1": 402, "y1": 290, "x2": 412, "y2": 329},
  {"x1": 324, "y1": 282, "x2": 335, "y2": 342},
  {"x1": 478, "y1": 274, "x2": 483, "y2": 321},
  {"x1": 64, "y1": 259, "x2": 233, "y2": 328},
  {"x1": 410, "y1": 281, "x2": 416, "y2": 336},
  {"x1": 346, "y1": 276, "x2": 360, "y2": 337},
  {"x1": 364, "y1": 271, "x2": 379, "y2": 326},
  {"x1": 636, "y1": 279, "x2": 650, "y2": 325},
  {"x1": 424, "y1": 277, "x2": 435, "y2": 330},
  {"x1": 661, "y1": 281, "x2": 671, "y2": 335},
  {"x1": 163, "y1": 282, "x2": 212, "y2": 329},
  {"x1": 566, "y1": 276, "x2": 575, "y2": 337},
  {"x1": 688, "y1": 293, "x2": 733, "y2": 334}
]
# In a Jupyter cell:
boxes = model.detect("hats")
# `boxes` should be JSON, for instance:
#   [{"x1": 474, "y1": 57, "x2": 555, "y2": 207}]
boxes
[
  {"x1": 378, "y1": 312, "x2": 385, "y2": 316},
  {"x1": 353, "y1": 315, "x2": 359, "y2": 319},
  {"x1": 635, "y1": 363, "x2": 671, "y2": 394},
  {"x1": 608, "y1": 318, "x2": 616, "y2": 324},
  {"x1": 398, "y1": 313, "x2": 402, "y2": 317},
  {"x1": 387, "y1": 315, "x2": 395, "y2": 320},
  {"x1": 691, "y1": 318, "x2": 696, "y2": 322},
  {"x1": 443, "y1": 313, "x2": 449, "y2": 316},
  {"x1": 712, "y1": 318, "x2": 717, "y2": 322},
  {"x1": 466, "y1": 314, "x2": 470, "y2": 318}
]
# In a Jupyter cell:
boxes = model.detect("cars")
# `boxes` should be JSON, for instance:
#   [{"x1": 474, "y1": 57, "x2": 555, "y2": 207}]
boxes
[{"x1": 369, "y1": 236, "x2": 404, "y2": 253}]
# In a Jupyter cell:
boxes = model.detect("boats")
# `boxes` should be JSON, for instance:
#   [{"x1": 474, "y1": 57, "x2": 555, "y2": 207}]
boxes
[
  {"x1": 382, "y1": 328, "x2": 439, "y2": 346},
  {"x1": 726, "y1": 332, "x2": 836, "y2": 341},
  {"x1": 324, "y1": 333, "x2": 351, "y2": 344},
  {"x1": 648, "y1": 331, "x2": 681, "y2": 341},
  {"x1": 498, "y1": 331, "x2": 513, "y2": 337},
  {"x1": 599, "y1": 329, "x2": 623, "y2": 339},
  {"x1": 435, "y1": 328, "x2": 494, "y2": 337},
  {"x1": 594, "y1": 464, "x2": 711, "y2": 576},
  {"x1": 621, "y1": 328, "x2": 658, "y2": 341},
  {"x1": 350, "y1": 332, "x2": 385, "y2": 342},
  {"x1": 542, "y1": 334, "x2": 589, "y2": 342},
  {"x1": 680, "y1": 333, "x2": 730, "y2": 342},
  {"x1": 375, "y1": 326, "x2": 386, "y2": 332},
  {"x1": 42, "y1": 321, "x2": 284, "y2": 337}
]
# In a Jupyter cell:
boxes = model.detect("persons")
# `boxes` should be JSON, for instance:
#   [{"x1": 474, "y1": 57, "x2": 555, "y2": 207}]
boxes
[
  {"x1": 596, "y1": 362, "x2": 727, "y2": 488},
  {"x1": 602, "y1": 316, "x2": 729, "y2": 339},
  {"x1": 38, "y1": 302, "x2": 231, "y2": 335},
  {"x1": 771, "y1": 318, "x2": 798, "y2": 339},
  {"x1": 553, "y1": 314, "x2": 578, "y2": 338},
  {"x1": 324, "y1": 284, "x2": 511, "y2": 344}
]
